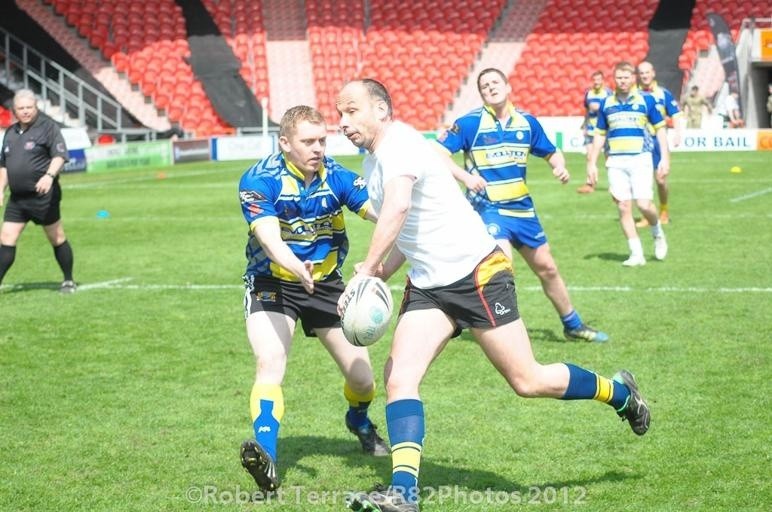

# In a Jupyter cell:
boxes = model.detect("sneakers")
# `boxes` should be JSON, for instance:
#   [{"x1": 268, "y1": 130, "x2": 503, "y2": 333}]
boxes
[
  {"x1": 346, "y1": 482, "x2": 420, "y2": 512},
  {"x1": 576, "y1": 185, "x2": 596, "y2": 193},
  {"x1": 623, "y1": 255, "x2": 646, "y2": 267},
  {"x1": 659, "y1": 208, "x2": 669, "y2": 225},
  {"x1": 563, "y1": 324, "x2": 608, "y2": 343},
  {"x1": 345, "y1": 411, "x2": 388, "y2": 455},
  {"x1": 61, "y1": 280, "x2": 77, "y2": 294},
  {"x1": 611, "y1": 368, "x2": 650, "y2": 435},
  {"x1": 239, "y1": 439, "x2": 279, "y2": 490},
  {"x1": 636, "y1": 216, "x2": 649, "y2": 227},
  {"x1": 653, "y1": 230, "x2": 667, "y2": 260}
]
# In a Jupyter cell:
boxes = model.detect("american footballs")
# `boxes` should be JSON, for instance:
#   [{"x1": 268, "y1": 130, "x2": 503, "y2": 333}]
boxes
[{"x1": 340, "y1": 273, "x2": 394, "y2": 347}]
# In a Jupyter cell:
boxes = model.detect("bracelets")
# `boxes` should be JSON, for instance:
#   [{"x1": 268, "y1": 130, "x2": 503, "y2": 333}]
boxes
[{"x1": 45, "y1": 171, "x2": 56, "y2": 180}]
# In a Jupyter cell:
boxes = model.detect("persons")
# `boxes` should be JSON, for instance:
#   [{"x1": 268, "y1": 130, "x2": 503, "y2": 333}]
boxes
[
  {"x1": 335, "y1": 78, "x2": 652, "y2": 512},
  {"x1": 238, "y1": 106, "x2": 393, "y2": 496},
  {"x1": 681, "y1": 85, "x2": 712, "y2": 128},
  {"x1": 766, "y1": 84, "x2": 772, "y2": 129},
  {"x1": 634, "y1": 61, "x2": 680, "y2": 229},
  {"x1": 714, "y1": 92, "x2": 742, "y2": 128},
  {"x1": 586, "y1": 62, "x2": 672, "y2": 266},
  {"x1": 0, "y1": 89, "x2": 78, "y2": 294},
  {"x1": 434, "y1": 68, "x2": 611, "y2": 343},
  {"x1": 575, "y1": 71, "x2": 615, "y2": 194}
]
